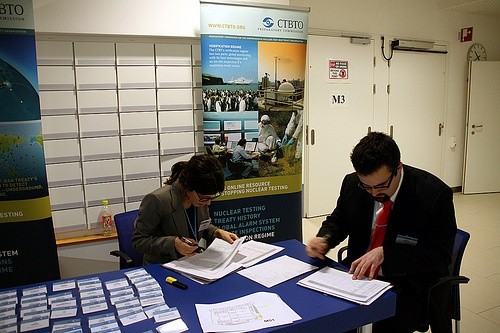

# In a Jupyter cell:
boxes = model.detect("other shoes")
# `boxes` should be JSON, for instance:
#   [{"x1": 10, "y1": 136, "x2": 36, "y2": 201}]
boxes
[{"x1": 270, "y1": 154, "x2": 277, "y2": 162}]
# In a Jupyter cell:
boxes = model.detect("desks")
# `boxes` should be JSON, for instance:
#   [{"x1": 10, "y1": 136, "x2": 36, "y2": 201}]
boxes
[{"x1": 0, "y1": 239, "x2": 397, "y2": 333}]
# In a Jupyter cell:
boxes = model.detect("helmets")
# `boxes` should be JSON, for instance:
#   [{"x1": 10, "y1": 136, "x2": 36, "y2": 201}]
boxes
[{"x1": 261, "y1": 114, "x2": 271, "y2": 122}]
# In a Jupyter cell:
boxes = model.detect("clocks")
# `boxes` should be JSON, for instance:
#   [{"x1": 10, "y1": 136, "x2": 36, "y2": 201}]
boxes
[{"x1": 467, "y1": 43, "x2": 487, "y2": 64}]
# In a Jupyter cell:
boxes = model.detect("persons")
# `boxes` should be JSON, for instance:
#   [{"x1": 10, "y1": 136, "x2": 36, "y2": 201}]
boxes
[
  {"x1": 212, "y1": 140, "x2": 226, "y2": 157},
  {"x1": 203, "y1": 88, "x2": 257, "y2": 113},
  {"x1": 131, "y1": 154, "x2": 240, "y2": 266},
  {"x1": 305, "y1": 132, "x2": 457, "y2": 333},
  {"x1": 233, "y1": 138, "x2": 260, "y2": 178},
  {"x1": 257, "y1": 115, "x2": 279, "y2": 145}
]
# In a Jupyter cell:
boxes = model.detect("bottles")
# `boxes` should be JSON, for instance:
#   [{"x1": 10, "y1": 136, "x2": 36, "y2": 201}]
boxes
[{"x1": 102, "y1": 200, "x2": 112, "y2": 236}]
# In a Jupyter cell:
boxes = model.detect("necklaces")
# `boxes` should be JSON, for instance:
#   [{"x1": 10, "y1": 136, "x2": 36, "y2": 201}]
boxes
[{"x1": 184, "y1": 206, "x2": 198, "y2": 243}]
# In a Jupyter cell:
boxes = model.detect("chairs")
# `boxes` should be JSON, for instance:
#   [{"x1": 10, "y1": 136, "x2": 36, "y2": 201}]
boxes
[
  {"x1": 109, "y1": 209, "x2": 143, "y2": 268},
  {"x1": 206, "y1": 147, "x2": 248, "y2": 179},
  {"x1": 338, "y1": 228, "x2": 471, "y2": 333}
]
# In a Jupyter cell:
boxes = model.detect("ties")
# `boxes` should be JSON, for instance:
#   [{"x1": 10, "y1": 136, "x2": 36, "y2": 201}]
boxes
[{"x1": 364, "y1": 200, "x2": 392, "y2": 279}]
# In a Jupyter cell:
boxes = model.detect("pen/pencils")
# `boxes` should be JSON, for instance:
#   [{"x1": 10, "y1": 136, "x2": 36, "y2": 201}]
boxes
[
  {"x1": 180, "y1": 236, "x2": 204, "y2": 254},
  {"x1": 310, "y1": 232, "x2": 333, "y2": 257}
]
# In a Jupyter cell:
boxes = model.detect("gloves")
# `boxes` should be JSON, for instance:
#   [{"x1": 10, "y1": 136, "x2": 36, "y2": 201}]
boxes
[
  {"x1": 282, "y1": 134, "x2": 289, "y2": 144},
  {"x1": 277, "y1": 142, "x2": 282, "y2": 150},
  {"x1": 286, "y1": 138, "x2": 295, "y2": 147}
]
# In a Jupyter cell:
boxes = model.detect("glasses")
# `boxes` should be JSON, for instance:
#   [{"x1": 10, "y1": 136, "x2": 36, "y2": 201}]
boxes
[
  {"x1": 195, "y1": 190, "x2": 223, "y2": 202},
  {"x1": 356, "y1": 166, "x2": 397, "y2": 190}
]
